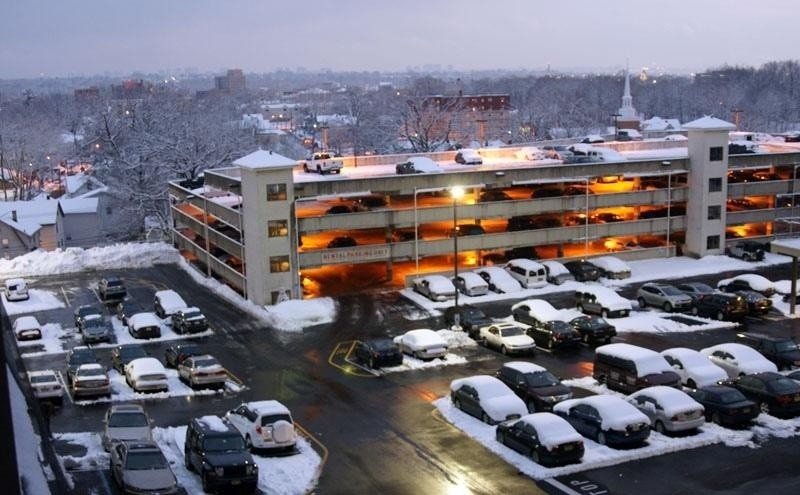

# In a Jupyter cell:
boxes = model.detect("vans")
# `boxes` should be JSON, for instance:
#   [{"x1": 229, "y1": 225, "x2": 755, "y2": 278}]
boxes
[{"x1": 592, "y1": 343, "x2": 683, "y2": 395}]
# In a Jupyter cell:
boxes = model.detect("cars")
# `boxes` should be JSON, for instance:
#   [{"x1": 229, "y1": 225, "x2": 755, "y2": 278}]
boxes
[
  {"x1": 177, "y1": 354, "x2": 228, "y2": 390},
  {"x1": 576, "y1": 285, "x2": 631, "y2": 319},
  {"x1": 717, "y1": 372, "x2": 800, "y2": 420},
  {"x1": 526, "y1": 320, "x2": 584, "y2": 349},
  {"x1": 164, "y1": 341, "x2": 203, "y2": 369},
  {"x1": 674, "y1": 281, "x2": 773, "y2": 322},
  {"x1": 496, "y1": 412, "x2": 585, "y2": 467},
  {"x1": 2, "y1": 277, "x2": 29, "y2": 302},
  {"x1": 717, "y1": 274, "x2": 776, "y2": 298},
  {"x1": 110, "y1": 439, "x2": 180, "y2": 495},
  {"x1": 664, "y1": 134, "x2": 688, "y2": 141},
  {"x1": 473, "y1": 267, "x2": 521, "y2": 294},
  {"x1": 116, "y1": 300, "x2": 144, "y2": 326},
  {"x1": 98, "y1": 276, "x2": 127, "y2": 300},
  {"x1": 682, "y1": 384, "x2": 760, "y2": 427},
  {"x1": 101, "y1": 404, "x2": 155, "y2": 452},
  {"x1": 479, "y1": 322, "x2": 537, "y2": 356},
  {"x1": 154, "y1": 289, "x2": 188, "y2": 319},
  {"x1": 777, "y1": 369, "x2": 800, "y2": 384},
  {"x1": 444, "y1": 304, "x2": 494, "y2": 336},
  {"x1": 26, "y1": 369, "x2": 64, "y2": 406},
  {"x1": 70, "y1": 363, "x2": 111, "y2": 400},
  {"x1": 14, "y1": 315, "x2": 42, "y2": 341},
  {"x1": 579, "y1": 135, "x2": 605, "y2": 143},
  {"x1": 127, "y1": 313, "x2": 161, "y2": 339},
  {"x1": 46, "y1": 167, "x2": 66, "y2": 181},
  {"x1": 624, "y1": 385, "x2": 706, "y2": 433},
  {"x1": 553, "y1": 394, "x2": 651, "y2": 445},
  {"x1": 78, "y1": 314, "x2": 112, "y2": 344},
  {"x1": 73, "y1": 304, "x2": 103, "y2": 327},
  {"x1": 511, "y1": 299, "x2": 557, "y2": 327},
  {"x1": 182, "y1": 171, "x2": 247, "y2": 275},
  {"x1": 699, "y1": 342, "x2": 778, "y2": 379},
  {"x1": 615, "y1": 128, "x2": 643, "y2": 141},
  {"x1": 659, "y1": 347, "x2": 729, "y2": 389},
  {"x1": 540, "y1": 261, "x2": 575, "y2": 285},
  {"x1": 517, "y1": 144, "x2": 604, "y2": 161},
  {"x1": 563, "y1": 260, "x2": 601, "y2": 282},
  {"x1": 454, "y1": 149, "x2": 483, "y2": 165},
  {"x1": 355, "y1": 338, "x2": 404, "y2": 370},
  {"x1": 451, "y1": 272, "x2": 489, "y2": 297},
  {"x1": 567, "y1": 315, "x2": 617, "y2": 344},
  {"x1": 587, "y1": 256, "x2": 632, "y2": 280},
  {"x1": 450, "y1": 375, "x2": 529, "y2": 425},
  {"x1": 111, "y1": 344, "x2": 152, "y2": 376},
  {"x1": 784, "y1": 131, "x2": 800, "y2": 142},
  {"x1": 636, "y1": 282, "x2": 693, "y2": 312},
  {"x1": 172, "y1": 307, "x2": 209, "y2": 334},
  {"x1": 726, "y1": 240, "x2": 765, "y2": 262},
  {"x1": 66, "y1": 344, "x2": 102, "y2": 383},
  {"x1": 413, "y1": 274, "x2": 460, "y2": 302},
  {"x1": 395, "y1": 156, "x2": 444, "y2": 174},
  {"x1": 124, "y1": 357, "x2": 169, "y2": 393},
  {"x1": 393, "y1": 328, "x2": 449, "y2": 361}
]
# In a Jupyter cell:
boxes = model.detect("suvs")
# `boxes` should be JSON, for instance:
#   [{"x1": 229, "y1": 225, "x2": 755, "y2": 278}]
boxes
[
  {"x1": 500, "y1": 259, "x2": 548, "y2": 289},
  {"x1": 736, "y1": 327, "x2": 800, "y2": 370},
  {"x1": 495, "y1": 361, "x2": 574, "y2": 413},
  {"x1": 303, "y1": 153, "x2": 343, "y2": 175},
  {"x1": 225, "y1": 399, "x2": 296, "y2": 454},
  {"x1": 184, "y1": 416, "x2": 259, "y2": 492}
]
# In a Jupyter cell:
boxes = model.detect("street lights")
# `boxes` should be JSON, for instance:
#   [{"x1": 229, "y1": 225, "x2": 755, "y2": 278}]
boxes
[
  {"x1": 22, "y1": 161, "x2": 36, "y2": 199},
  {"x1": 731, "y1": 108, "x2": 745, "y2": 130},
  {"x1": 157, "y1": 76, "x2": 178, "y2": 104},
  {"x1": 46, "y1": 152, "x2": 54, "y2": 181},
  {"x1": 271, "y1": 113, "x2": 283, "y2": 129}
]
